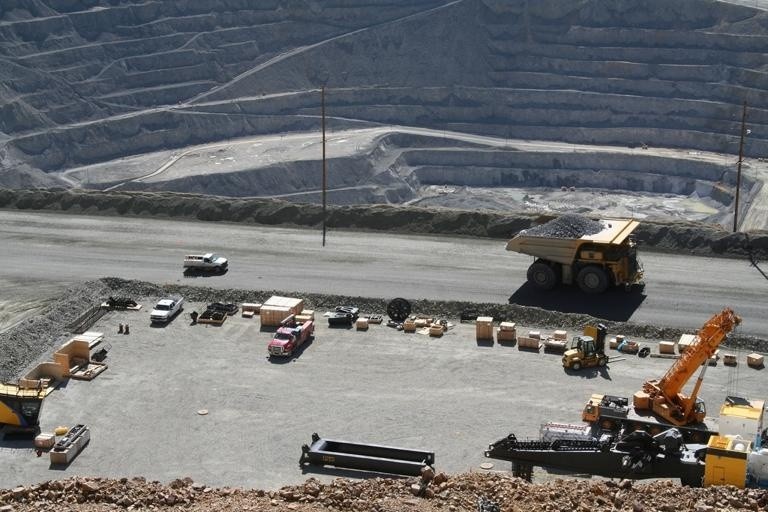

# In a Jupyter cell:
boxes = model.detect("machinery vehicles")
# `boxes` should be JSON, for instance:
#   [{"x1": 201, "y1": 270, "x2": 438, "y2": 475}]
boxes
[
  {"x1": 563, "y1": 323, "x2": 626, "y2": 372},
  {"x1": 582, "y1": 309, "x2": 750, "y2": 440},
  {"x1": 505, "y1": 215, "x2": 645, "y2": 296}
]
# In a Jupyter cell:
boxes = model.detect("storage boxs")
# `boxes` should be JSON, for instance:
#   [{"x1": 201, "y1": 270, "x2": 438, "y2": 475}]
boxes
[
  {"x1": 403, "y1": 317, "x2": 446, "y2": 338},
  {"x1": 72, "y1": 425, "x2": 93, "y2": 442},
  {"x1": 610, "y1": 334, "x2": 695, "y2": 359},
  {"x1": 475, "y1": 316, "x2": 567, "y2": 352},
  {"x1": 705, "y1": 349, "x2": 763, "y2": 370},
  {"x1": 64, "y1": 436, "x2": 88, "y2": 450},
  {"x1": 355, "y1": 316, "x2": 369, "y2": 330},
  {"x1": 241, "y1": 296, "x2": 314, "y2": 327},
  {"x1": 34, "y1": 430, "x2": 55, "y2": 450},
  {"x1": 43, "y1": 442, "x2": 76, "y2": 465}
]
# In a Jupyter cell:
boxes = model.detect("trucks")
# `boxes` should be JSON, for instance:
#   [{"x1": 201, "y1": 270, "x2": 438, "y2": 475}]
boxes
[{"x1": 267, "y1": 314, "x2": 313, "y2": 358}]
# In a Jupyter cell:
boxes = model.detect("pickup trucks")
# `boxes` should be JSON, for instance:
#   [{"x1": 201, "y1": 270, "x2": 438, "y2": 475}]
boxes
[
  {"x1": 150, "y1": 296, "x2": 184, "y2": 323},
  {"x1": 183, "y1": 252, "x2": 227, "y2": 274}
]
